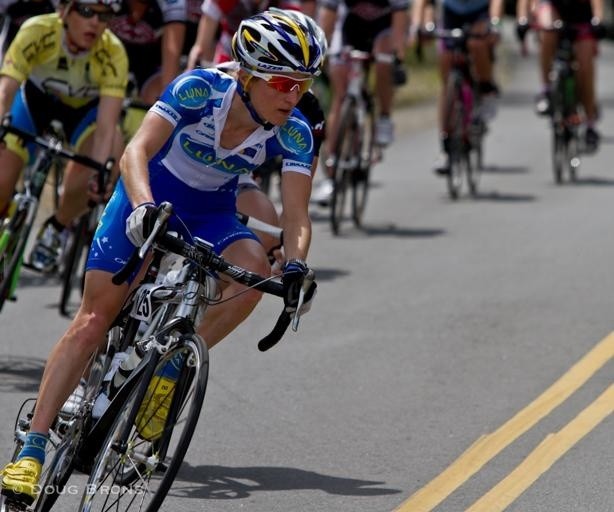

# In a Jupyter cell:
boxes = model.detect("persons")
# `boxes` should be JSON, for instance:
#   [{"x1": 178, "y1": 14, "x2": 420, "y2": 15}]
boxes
[
  {"x1": 0, "y1": 6, "x2": 327, "y2": 500},
  {"x1": 0, "y1": 1, "x2": 127, "y2": 273},
  {"x1": 0, "y1": 1, "x2": 613, "y2": 205}
]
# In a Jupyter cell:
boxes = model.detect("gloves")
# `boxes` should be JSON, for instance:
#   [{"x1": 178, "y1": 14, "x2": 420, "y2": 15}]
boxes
[
  {"x1": 280, "y1": 258, "x2": 317, "y2": 319},
  {"x1": 125, "y1": 203, "x2": 168, "y2": 247}
]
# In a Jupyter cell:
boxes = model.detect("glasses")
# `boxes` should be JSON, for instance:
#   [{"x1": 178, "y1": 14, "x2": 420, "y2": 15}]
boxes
[
  {"x1": 238, "y1": 64, "x2": 314, "y2": 96},
  {"x1": 72, "y1": 3, "x2": 111, "y2": 20}
]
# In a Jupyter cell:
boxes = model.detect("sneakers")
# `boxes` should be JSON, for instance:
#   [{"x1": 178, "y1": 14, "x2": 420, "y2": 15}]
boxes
[
  {"x1": 373, "y1": 117, "x2": 394, "y2": 145},
  {"x1": 0, "y1": 456, "x2": 42, "y2": 506},
  {"x1": 28, "y1": 215, "x2": 69, "y2": 273},
  {"x1": 135, "y1": 374, "x2": 177, "y2": 441}
]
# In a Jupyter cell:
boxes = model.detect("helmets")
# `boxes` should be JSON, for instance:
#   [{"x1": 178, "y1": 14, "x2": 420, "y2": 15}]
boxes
[{"x1": 231, "y1": 8, "x2": 328, "y2": 78}]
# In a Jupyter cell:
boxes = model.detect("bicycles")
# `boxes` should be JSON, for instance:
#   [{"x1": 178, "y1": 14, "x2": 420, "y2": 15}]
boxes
[
  {"x1": 518, "y1": 18, "x2": 612, "y2": 182},
  {"x1": 1, "y1": 201, "x2": 318, "y2": 511},
  {"x1": 412, "y1": 21, "x2": 504, "y2": 199},
  {"x1": 0, "y1": 94, "x2": 163, "y2": 312},
  {"x1": 325, "y1": 43, "x2": 405, "y2": 231}
]
